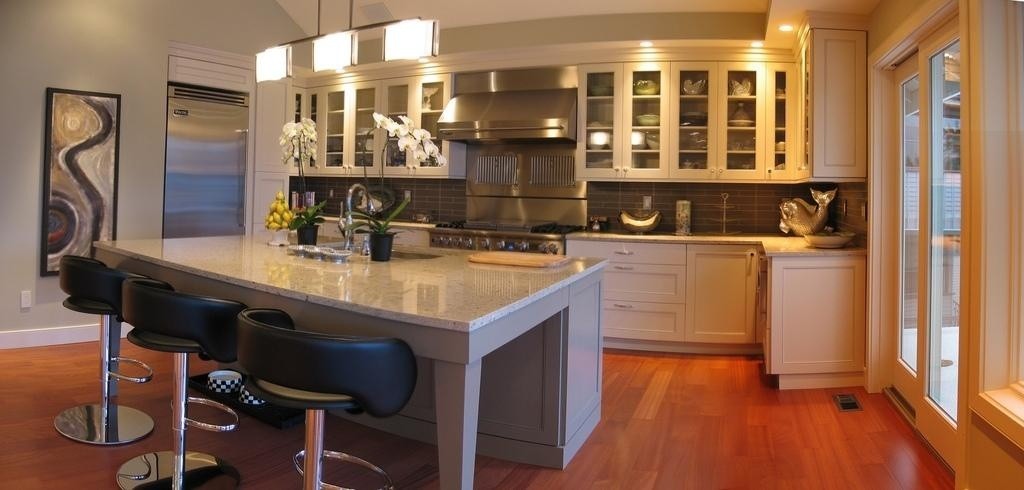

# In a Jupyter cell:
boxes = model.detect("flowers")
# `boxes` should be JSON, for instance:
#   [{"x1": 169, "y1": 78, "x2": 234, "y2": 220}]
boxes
[
  {"x1": 279, "y1": 117, "x2": 327, "y2": 230},
  {"x1": 344, "y1": 112, "x2": 447, "y2": 234}
]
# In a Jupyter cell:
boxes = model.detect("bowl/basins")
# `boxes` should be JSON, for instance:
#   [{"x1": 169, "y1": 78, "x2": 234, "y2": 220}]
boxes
[{"x1": 588, "y1": 74, "x2": 786, "y2": 171}]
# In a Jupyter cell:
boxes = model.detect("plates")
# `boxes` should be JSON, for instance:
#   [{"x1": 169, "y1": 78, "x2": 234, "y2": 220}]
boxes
[{"x1": 803, "y1": 232, "x2": 857, "y2": 248}]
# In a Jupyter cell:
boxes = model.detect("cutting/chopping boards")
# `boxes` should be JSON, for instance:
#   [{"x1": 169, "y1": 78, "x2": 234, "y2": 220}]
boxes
[{"x1": 469, "y1": 250, "x2": 575, "y2": 269}]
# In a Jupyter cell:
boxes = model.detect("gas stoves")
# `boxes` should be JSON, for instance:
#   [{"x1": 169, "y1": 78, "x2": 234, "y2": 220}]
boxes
[{"x1": 434, "y1": 217, "x2": 578, "y2": 255}]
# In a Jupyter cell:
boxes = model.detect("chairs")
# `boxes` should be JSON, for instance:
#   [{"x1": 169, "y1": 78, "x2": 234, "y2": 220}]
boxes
[
  {"x1": 53, "y1": 255, "x2": 153, "y2": 444},
  {"x1": 239, "y1": 308, "x2": 417, "y2": 490},
  {"x1": 116, "y1": 279, "x2": 248, "y2": 490}
]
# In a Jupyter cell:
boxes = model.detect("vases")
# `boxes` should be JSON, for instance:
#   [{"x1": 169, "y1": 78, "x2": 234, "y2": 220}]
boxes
[
  {"x1": 297, "y1": 225, "x2": 319, "y2": 245},
  {"x1": 370, "y1": 233, "x2": 394, "y2": 261}
]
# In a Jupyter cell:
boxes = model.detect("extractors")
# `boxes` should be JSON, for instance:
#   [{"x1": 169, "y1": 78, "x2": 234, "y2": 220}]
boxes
[{"x1": 438, "y1": 63, "x2": 579, "y2": 145}]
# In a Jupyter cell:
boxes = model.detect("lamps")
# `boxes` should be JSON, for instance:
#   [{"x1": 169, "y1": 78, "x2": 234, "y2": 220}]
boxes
[{"x1": 255, "y1": 1, "x2": 439, "y2": 81}]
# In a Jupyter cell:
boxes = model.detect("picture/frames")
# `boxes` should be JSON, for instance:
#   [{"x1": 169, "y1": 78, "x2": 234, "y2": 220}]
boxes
[{"x1": 39, "y1": 86, "x2": 122, "y2": 277}]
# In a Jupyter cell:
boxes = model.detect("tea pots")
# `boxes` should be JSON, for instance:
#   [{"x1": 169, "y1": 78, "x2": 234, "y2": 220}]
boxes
[{"x1": 589, "y1": 216, "x2": 603, "y2": 231}]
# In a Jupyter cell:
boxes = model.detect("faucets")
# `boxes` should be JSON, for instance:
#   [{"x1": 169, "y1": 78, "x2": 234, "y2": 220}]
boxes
[{"x1": 338, "y1": 184, "x2": 375, "y2": 251}]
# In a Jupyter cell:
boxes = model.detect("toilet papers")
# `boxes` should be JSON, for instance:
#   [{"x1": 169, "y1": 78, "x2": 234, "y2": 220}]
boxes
[{"x1": 674, "y1": 199, "x2": 692, "y2": 235}]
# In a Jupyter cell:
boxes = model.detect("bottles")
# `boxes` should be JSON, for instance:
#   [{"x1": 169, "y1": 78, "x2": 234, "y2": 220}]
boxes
[{"x1": 360, "y1": 235, "x2": 369, "y2": 256}]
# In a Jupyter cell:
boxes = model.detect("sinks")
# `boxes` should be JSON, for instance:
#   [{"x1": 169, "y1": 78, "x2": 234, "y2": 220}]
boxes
[
  {"x1": 391, "y1": 250, "x2": 440, "y2": 261},
  {"x1": 319, "y1": 241, "x2": 366, "y2": 252}
]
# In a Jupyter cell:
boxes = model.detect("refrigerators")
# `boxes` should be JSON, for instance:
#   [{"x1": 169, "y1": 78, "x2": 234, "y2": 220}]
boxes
[{"x1": 164, "y1": 85, "x2": 247, "y2": 241}]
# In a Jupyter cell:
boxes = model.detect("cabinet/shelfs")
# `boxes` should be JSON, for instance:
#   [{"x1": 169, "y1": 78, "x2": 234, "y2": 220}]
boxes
[
  {"x1": 255, "y1": 82, "x2": 321, "y2": 178},
  {"x1": 687, "y1": 244, "x2": 763, "y2": 352},
  {"x1": 566, "y1": 241, "x2": 686, "y2": 354},
  {"x1": 381, "y1": 71, "x2": 466, "y2": 178},
  {"x1": 574, "y1": 28, "x2": 867, "y2": 182},
  {"x1": 321, "y1": 79, "x2": 381, "y2": 177},
  {"x1": 764, "y1": 255, "x2": 868, "y2": 390}
]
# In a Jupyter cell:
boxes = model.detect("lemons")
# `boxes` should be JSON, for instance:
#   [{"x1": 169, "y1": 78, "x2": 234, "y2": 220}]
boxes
[{"x1": 264, "y1": 190, "x2": 295, "y2": 230}]
